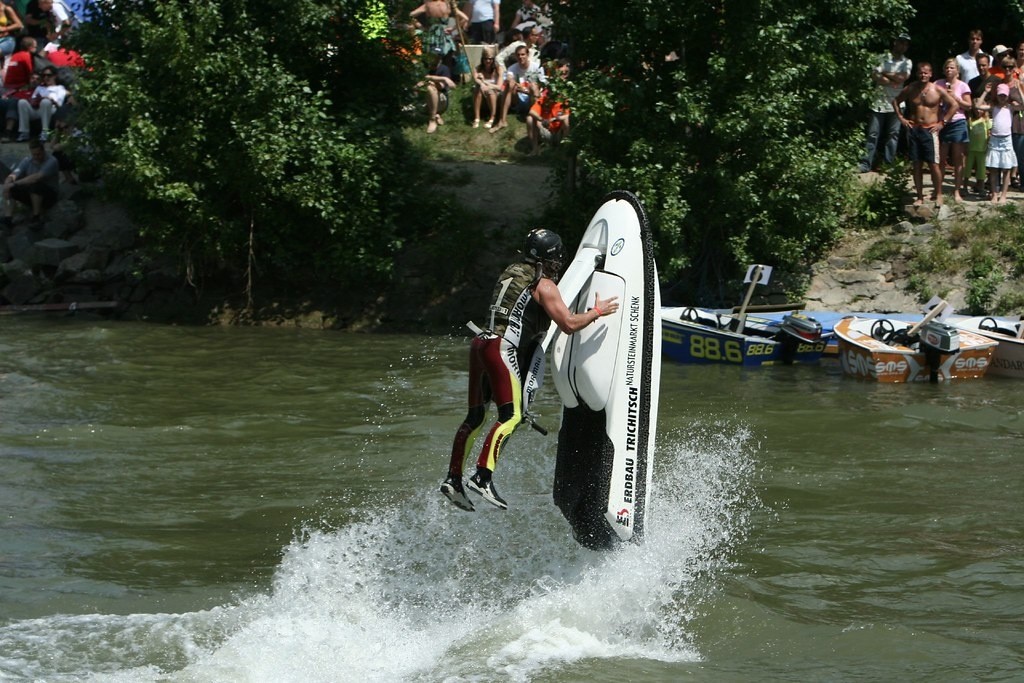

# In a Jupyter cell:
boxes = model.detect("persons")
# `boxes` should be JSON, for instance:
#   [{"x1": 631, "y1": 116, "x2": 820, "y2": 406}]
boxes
[
  {"x1": 962, "y1": 98, "x2": 993, "y2": 196},
  {"x1": 439, "y1": 229, "x2": 619, "y2": 512},
  {"x1": 933, "y1": 58, "x2": 971, "y2": 203},
  {"x1": 975, "y1": 82, "x2": 1024, "y2": 204},
  {"x1": 470, "y1": 0, "x2": 572, "y2": 156},
  {"x1": 0, "y1": 140, "x2": 60, "y2": 236},
  {"x1": 405, "y1": 47, "x2": 457, "y2": 133},
  {"x1": 1015, "y1": 42, "x2": 1024, "y2": 82},
  {"x1": 1001, "y1": 56, "x2": 1024, "y2": 188},
  {"x1": 968, "y1": 53, "x2": 1003, "y2": 124},
  {"x1": 956, "y1": 29, "x2": 993, "y2": 84},
  {"x1": 854, "y1": 33, "x2": 912, "y2": 173},
  {"x1": 0, "y1": 0, "x2": 93, "y2": 177},
  {"x1": 462, "y1": 0, "x2": 502, "y2": 45},
  {"x1": 891, "y1": 61, "x2": 959, "y2": 206},
  {"x1": 987, "y1": 45, "x2": 1013, "y2": 79},
  {"x1": 409, "y1": 0, "x2": 469, "y2": 57}
]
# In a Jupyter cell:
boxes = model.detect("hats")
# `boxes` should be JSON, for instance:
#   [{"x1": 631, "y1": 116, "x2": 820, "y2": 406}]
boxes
[
  {"x1": 897, "y1": 32, "x2": 911, "y2": 41},
  {"x1": 992, "y1": 45, "x2": 1013, "y2": 58}
]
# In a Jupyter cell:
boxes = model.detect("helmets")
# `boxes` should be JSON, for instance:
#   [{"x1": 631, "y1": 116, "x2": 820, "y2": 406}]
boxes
[{"x1": 523, "y1": 228, "x2": 563, "y2": 263}]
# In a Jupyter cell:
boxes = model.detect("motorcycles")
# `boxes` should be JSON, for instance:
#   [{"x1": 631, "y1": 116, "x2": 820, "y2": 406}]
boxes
[{"x1": 522, "y1": 190, "x2": 662, "y2": 551}]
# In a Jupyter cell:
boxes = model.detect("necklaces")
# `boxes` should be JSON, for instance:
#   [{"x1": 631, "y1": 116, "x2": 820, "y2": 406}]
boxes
[{"x1": 919, "y1": 84, "x2": 931, "y2": 97}]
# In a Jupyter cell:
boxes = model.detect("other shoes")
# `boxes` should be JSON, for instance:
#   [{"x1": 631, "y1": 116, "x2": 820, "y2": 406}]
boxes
[
  {"x1": 0, "y1": 216, "x2": 13, "y2": 225},
  {"x1": 473, "y1": 118, "x2": 480, "y2": 129},
  {"x1": 40, "y1": 132, "x2": 48, "y2": 141},
  {"x1": 483, "y1": 119, "x2": 494, "y2": 129},
  {"x1": 852, "y1": 166, "x2": 861, "y2": 174},
  {"x1": 427, "y1": 114, "x2": 444, "y2": 134},
  {"x1": 30, "y1": 215, "x2": 40, "y2": 227},
  {"x1": 16, "y1": 133, "x2": 31, "y2": 142}
]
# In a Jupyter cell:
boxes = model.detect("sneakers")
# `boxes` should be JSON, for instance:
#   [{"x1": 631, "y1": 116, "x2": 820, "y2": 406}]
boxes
[
  {"x1": 465, "y1": 473, "x2": 508, "y2": 510},
  {"x1": 440, "y1": 476, "x2": 476, "y2": 512}
]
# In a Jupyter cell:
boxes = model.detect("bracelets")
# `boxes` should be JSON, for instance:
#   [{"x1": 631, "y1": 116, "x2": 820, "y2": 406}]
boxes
[
  {"x1": 942, "y1": 119, "x2": 947, "y2": 126},
  {"x1": 594, "y1": 308, "x2": 601, "y2": 315}
]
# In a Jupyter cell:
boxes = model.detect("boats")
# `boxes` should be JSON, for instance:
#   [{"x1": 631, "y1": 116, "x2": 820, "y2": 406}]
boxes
[
  {"x1": 832, "y1": 315, "x2": 999, "y2": 384},
  {"x1": 660, "y1": 306, "x2": 834, "y2": 367},
  {"x1": 921, "y1": 312, "x2": 1024, "y2": 381}
]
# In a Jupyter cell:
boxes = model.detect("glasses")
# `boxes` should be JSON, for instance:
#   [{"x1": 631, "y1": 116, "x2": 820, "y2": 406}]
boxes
[{"x1": 40, "y1": 74, "x2": 54, "y2": 78}]
